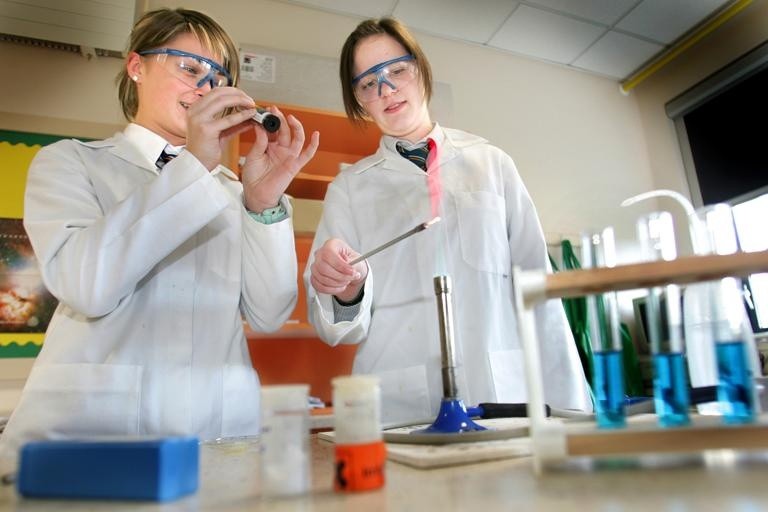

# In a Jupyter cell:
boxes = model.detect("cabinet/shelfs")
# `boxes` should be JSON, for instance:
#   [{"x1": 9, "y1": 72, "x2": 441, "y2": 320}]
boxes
[{"x1": 231, "y1": 100, "x2": 384, "y2": 434}]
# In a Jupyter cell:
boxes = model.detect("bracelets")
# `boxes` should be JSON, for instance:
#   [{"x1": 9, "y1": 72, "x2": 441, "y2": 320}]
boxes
[{"x1": 240, "y1": 204, "x2": 290, "y2": 225}]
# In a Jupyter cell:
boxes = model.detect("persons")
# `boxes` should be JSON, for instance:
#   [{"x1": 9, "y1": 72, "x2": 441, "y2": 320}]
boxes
[
  {"x1": 299, "y1": 14, "x2": 599, "y2": 424},
  {"x1": 1, "y1": 6, "x2": 323, "y2": 484}
]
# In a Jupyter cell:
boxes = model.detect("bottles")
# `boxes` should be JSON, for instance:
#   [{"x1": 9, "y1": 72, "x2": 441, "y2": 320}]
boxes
[
  {"x1": 583, "y1": 225, "x2": 628, "y2": 430},
  {"x1": 635, "y1": 210, "x2": 693, "y2": 427},
  {"x1": 694, "y1": 203, "x2": 760, "y2": 423},
  {"x1": 260, "y1": 373, "x2": 386, "y2": 499}
]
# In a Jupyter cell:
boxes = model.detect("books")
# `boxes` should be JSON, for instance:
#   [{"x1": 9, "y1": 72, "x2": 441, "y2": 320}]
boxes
[{"x1": 316, "y1": 417, "x2": 563, "y2": 471}]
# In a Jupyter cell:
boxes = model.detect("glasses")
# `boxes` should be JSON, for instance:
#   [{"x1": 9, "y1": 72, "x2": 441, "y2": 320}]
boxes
[
  {"x1": 139, "y1": 49, "x2": 235, "y2": 92},
  {"x1": 349, "y1": 54, "x2": 419, "y2": 105}
]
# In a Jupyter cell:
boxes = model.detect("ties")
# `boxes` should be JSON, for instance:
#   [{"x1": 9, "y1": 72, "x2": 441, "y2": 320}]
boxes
[
  {"x1": 157, "y1": 150, "x2": 176, "y2": 163},
  {"x1": 397, "y1": 144, "x2": 430, "y2": 170}
]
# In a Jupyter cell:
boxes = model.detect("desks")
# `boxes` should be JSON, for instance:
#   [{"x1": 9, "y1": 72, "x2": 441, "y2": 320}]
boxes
[{"x1": 1, "y1": 412, "x2": 768, "y2": 512}]
